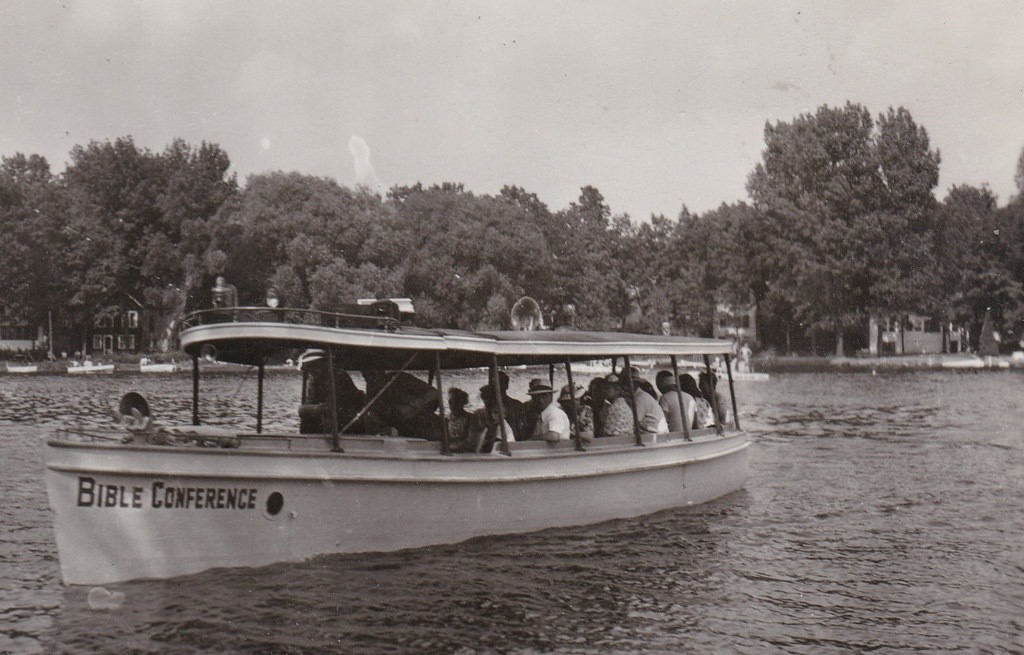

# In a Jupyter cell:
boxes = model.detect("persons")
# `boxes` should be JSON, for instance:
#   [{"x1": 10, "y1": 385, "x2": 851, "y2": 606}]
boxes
[
  {"x1": 698, "y1": 372, "x2": 727, "y2": 425},
  {"x1": 740, "y1": 344, "x2": 753, "y2": 373},
  {"x1": 296, "y1": 349, "x2": 366, "y2": 435},
  {"x1": 679, "y1": 374, "x2": 715, "y2": 430},
  {"x1": 588, "y1": 378, "x2": 612, "y2": 437},
  {"x1": 74, "y1": 350, "x2": 80, "y2": 360},
  {"x1": 61, "y1": 350, "x2": 67, "y2": 360},
  {"x1": 468, "y1": 385, "x2": 493, "y2": 453},
  {"x1": 527, "y1": 378, "x2": 570, "y2": 441},
  {"x1": 488, "y1": 400, "x2": 515, "y2": 442},
  {"x1": 604, "y1": 374, "x2": 634, "y2": 436},
  {"x1": 656, "y1": 370, "x2": 696, "y2": 432},
  {"x1": 619, "y1": 367, "x2": 669, "y2": 435},
  {"x1": 497, "y1": 370, "x2": 522, "y2": 441},
  {"x1": 558, "y1": 385, "x2": 595, "y2": 442},
  {"x1": 48, "y1": 351, "x2": 55, "y2": 363},
  {"x1": 83, "y1": 355, "x2": 93, "y2": 366},
  {"x1": 357, "y1": 349, "x2": 448, "y2": 441},
  {"x1": 446, "y1": 387, "x2": 475, "y2": 454}
]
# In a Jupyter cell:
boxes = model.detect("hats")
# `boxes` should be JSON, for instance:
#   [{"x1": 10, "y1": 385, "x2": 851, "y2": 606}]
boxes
[
  {"x1": 558, "y1": 381, "x2": 585, "y2": 400},
  {"x1": 526, "y1": 377, "x2": 558, "y2": 394},
  {"x1": 617, "y1": 366, "x2": 643, "y2": 383}
]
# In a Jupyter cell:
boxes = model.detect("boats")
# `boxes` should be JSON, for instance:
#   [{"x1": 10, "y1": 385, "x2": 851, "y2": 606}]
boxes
[
  {"x1": 140, "y1": 358, "x2": 179, "y2": 373},
  {"x1": 67, "y1": 358, "x2": 114, "y2": 373},
  {"x1": 5, "y1": 362, "x2": 38, "y2": 373},
  {"x1": 40, "y1": 312, "x2": 750, "y2": 586},
  {"x1": 570, "y1": 359, "x2": 620, "y2": 376},
  {"x1": 712, "y1": 359, "x2": 768, "y2": 382}
]
